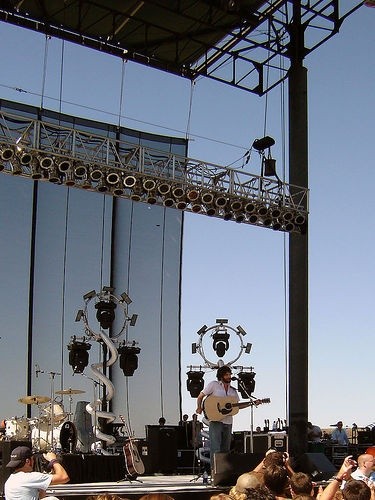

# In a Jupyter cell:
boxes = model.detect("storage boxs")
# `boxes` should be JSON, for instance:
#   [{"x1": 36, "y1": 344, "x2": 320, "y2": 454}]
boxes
[
  {"x1": 325, "y1": 446, "x2": 348, "y2": 466},
  {"x1": 177, "y1": 450, "x2": 200, "y2": 469},
  {"x1": 244, "y1": 434, "x2": 288, "y2": 455}
]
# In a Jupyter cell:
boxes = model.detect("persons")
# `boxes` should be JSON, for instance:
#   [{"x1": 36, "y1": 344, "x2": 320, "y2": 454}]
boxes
[
  {"x1": 4, "y1": 446, "x2": 70, "y2": 500},
  {"x1": 190, "y1": 414, "x2": 199, "y2": 422},
  {"x1": 256, "y1": 427, "x2": 261, "y2": 432},
  {"x1": 210, "y1": 449, "x2": 317, "y2": 500},
  {"x1": 196, "y1": 366, "x2": 262, "y2": 472},
  {"x1": 316, "y1": 446, "x2": 375, "y2": 500},
  {"x1": 151, "y1": 417, "x2": 166, "y2": 476},
  {"x1": 87, "y1": 492, "x2": 174, "y2": 500},
  {"x1": 307, "y1": 422, "x2": 322, "y2": 437},
  {"x1": 332, "y1": 420, "x2": 350, "y2": 445},
  {"x1": 178, "y1": 414, "x2": 188, "y2": 425}
]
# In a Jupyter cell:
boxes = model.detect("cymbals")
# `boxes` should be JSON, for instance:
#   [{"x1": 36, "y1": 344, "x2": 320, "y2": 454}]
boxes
[
  {"x1": 18, "y1": 395, "x2": 50, "y2": 405},
  {"x1": 55, "y1": 389, "x2": 86, "y2": 394}
]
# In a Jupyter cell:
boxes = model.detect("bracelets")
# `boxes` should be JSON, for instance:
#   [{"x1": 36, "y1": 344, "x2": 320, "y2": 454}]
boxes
[
  {"x1": 334, "y1": 478, "x2": 342, "y2": 483},
  {"x1": 51, "y1": 459, "x2": 59, "y2": 467}
]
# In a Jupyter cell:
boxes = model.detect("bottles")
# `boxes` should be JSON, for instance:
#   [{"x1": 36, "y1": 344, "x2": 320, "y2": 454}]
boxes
[
  {"x1": 274, "y1": 418, "x2": 284, "y2": 431},
  {"x1": 203, "y1": 471, "x2": 208, "y2": 485}
]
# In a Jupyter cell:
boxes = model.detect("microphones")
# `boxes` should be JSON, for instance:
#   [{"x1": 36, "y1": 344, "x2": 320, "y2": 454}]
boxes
[{"x1": 231, "y1": 377, "x2": 240, "y2": 381}]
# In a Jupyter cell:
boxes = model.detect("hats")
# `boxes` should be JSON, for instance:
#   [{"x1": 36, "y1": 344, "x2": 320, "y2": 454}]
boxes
[{"x1": 5, "y1": 446, "x2": 33, "y2": 467}]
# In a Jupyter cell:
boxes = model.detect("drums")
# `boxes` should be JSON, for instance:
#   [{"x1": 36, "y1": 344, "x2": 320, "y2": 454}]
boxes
[
  {"x1": 5, "y1": 417, "x2": 30, "y2": 440},
  {"x1": 29, "y1": 417, "x2": 42, "y2": 429},
  {"x1": 32, "y1": 421, "x2": 78, "y2": 452},
  {"x1": 44, "y1": 403, "x2": 66, "y2": 421}
]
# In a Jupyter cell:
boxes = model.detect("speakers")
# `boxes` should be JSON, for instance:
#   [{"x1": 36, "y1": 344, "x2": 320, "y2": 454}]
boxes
[
  {"x1": 210, "y1": 452, "x2": 263, "y2": 485},
  {"x1": 294, "y1": 452, "x2": 337, "y2": 482},
  {"x1": 0, "y1": 440, "x2": 32, "y2": 493}
]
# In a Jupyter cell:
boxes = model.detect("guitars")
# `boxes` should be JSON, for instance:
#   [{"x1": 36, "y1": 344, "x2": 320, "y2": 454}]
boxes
[
  {"x1": 118, "y1": 414, "x2": 145, "y2": 477},
  {"x1": 203, "y1": 395, "x2": 270, "y2": 422}
]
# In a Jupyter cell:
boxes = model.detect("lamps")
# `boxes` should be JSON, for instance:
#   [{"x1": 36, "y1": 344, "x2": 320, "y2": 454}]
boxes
[
  {"x1": 0, "y1": 137, "x2": 305, "y2": 235},
  {"x1": 67, "y1": 335, "x2": 92, "y2": 373},
  {"x1": 237, "y1": 366, "x2": 256, "y2": 399},
  {"x1": 186, "y1": 365, "x2": 205, "y2": 398},
  {"x1": 95, "y1": 300, "x2": 117, "y2": 329},
  {"x1": 213, "y1": 331, "x2": 230, "y2": 357},
  {"x1": 117, "y1": 340, "x2": 141, "y2": 376}
]
